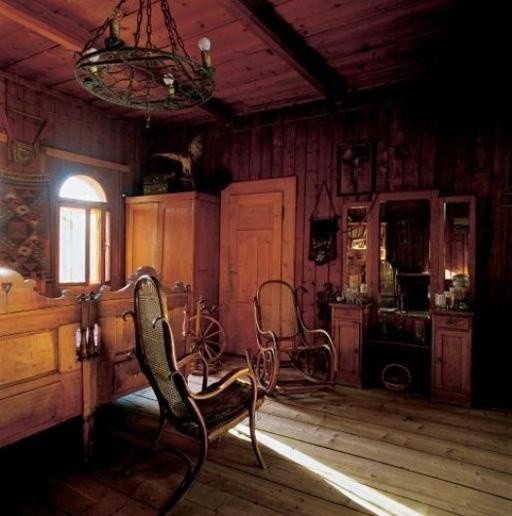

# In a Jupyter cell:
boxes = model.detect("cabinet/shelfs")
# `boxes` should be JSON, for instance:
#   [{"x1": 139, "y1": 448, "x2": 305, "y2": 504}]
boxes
[
  {"x1": 327, "y1": 301, "x2": 373, "y2": 388},
  {"x1": 125, "y1": 192, "x2": 220, "y2": 350},
  {"x1": 431, "y1": 311, "x2": 476, "y2": 408}
]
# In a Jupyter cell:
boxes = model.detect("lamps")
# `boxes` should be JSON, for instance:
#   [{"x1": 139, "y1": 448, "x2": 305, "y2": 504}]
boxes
[{"x1": 72, "y1": 1, "x2": 216, "y2": 129}]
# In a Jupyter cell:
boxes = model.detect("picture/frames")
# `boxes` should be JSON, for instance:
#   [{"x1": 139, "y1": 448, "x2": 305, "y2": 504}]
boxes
[{"x1": 337, "y1": 137, "x2": 376, "y2": 196}]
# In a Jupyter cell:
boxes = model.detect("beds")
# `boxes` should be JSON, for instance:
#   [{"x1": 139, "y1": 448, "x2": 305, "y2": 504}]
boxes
[
  {"x1": 88, "y1": 266, "x2": 193, "y2": 471},
  {"x1": 0, "y1": 267, "x2": 88, "y2": 472}
]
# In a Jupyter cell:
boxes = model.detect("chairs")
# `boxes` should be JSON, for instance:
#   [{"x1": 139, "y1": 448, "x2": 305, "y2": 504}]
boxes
[
  {"x1": 379, "y1": 262, "x2": 406, "y2": 313},
  {"x1": 244, "y1": 279, "x2": 339, "y2": 394},
  {"x1": 122, "y1": 273, "x2": 267, "y2": 515}
]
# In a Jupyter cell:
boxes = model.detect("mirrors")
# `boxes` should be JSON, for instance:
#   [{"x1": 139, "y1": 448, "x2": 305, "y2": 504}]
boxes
[
  {"x1": 445, "y1": 202, "x2": 470, "y2": 291},
  {"x1": 379, "y1": 199, "x2": 431, "y2": 316},
  {"x1": 347, "y1": 208, "x2": 368, "y2": 299}
]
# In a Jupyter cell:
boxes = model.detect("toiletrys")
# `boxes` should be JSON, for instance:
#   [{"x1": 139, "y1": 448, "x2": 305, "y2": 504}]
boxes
[{"x1": 445, "y1": 293, "x2": 452, "y2": 310}]
